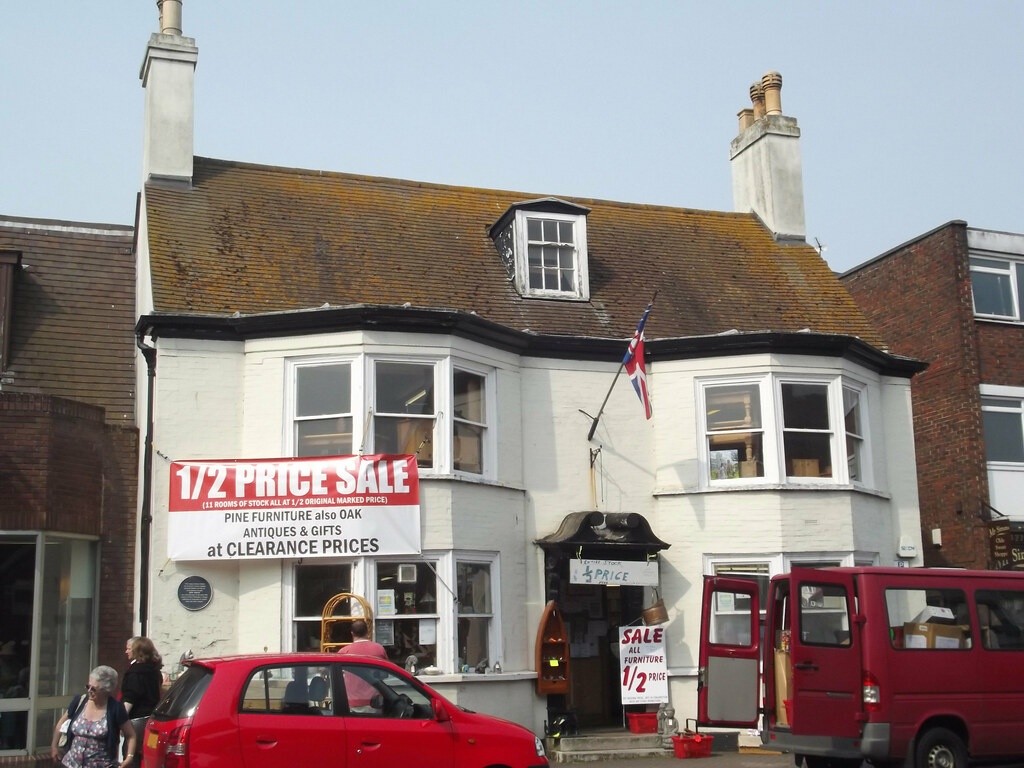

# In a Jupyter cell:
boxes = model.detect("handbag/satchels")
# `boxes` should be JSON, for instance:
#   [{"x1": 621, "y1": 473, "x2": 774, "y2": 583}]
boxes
[{"x1": 58, "y1": 694, "x2": 87, "y2": 747}]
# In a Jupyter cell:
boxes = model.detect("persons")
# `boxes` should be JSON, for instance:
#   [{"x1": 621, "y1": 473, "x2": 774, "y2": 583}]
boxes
[
  {"x1": 120, "y1": 637, "x2": 164, "y2": 768},
  {"x1": 125, "y1": 636, "x2": 161, "y2": 668},
  {"x1": 338, "y1": 619, "x2": 389, "y2": 713},
  {"x1": 51, "y1": 665, "x2": 137, "y2": 768}
]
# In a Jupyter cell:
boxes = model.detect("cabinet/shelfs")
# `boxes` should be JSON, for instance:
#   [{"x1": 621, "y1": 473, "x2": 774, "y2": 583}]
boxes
[
  {"x1": 535, "y1": 601, "x2": 571, "y2": 695},
  {"x1": 787, "y1": 459, "x2": 820, "y2": 476}
]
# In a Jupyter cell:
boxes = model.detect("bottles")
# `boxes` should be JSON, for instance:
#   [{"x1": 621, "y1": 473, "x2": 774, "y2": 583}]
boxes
[{"x1": 493, "y1": 661, "x2": 501, "y2": 674}]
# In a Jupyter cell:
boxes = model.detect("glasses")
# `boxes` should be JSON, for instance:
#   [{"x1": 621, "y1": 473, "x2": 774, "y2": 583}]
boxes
[{"x1": 84, "y1": 684, "x2": 106, "y2": 693}]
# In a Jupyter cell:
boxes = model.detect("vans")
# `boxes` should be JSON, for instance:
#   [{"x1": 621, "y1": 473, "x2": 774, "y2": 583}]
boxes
[{"x1": 698, "y1": 566, "x2": 1024, "y2": 768}]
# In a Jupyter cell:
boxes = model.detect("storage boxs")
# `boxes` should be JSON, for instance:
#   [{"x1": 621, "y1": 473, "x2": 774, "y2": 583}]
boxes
[
  {"x1": 886, "y1": 606, "x2": 969, "y2": 650},
  {"x1": 775, "y1": 630, "x2": 793, "y2": 729},
  {"x1": 738, "y1": 731, "x2": 782, "y2": 755}
]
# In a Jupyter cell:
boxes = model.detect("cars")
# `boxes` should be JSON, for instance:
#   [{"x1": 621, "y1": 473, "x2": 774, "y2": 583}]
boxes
[{"x1": 142, "y1": 652, "x2": 549, "y2": 768}]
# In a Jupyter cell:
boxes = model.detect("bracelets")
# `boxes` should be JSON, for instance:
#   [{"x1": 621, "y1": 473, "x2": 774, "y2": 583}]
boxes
[{"x1": 127, "y1": 754, "x2": 134, "y2": 757}]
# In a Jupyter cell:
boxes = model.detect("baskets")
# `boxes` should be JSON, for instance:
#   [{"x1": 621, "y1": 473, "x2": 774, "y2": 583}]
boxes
[
  {"x1": 672, "y1": 719, "x2": 715, "y2": 759},
  {"x1": 626, "y1": 712, "x2": 659, "y2": 733}
]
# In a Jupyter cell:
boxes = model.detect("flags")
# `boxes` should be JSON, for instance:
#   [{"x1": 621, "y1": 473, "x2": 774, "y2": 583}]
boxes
[{"x1": 622, "y1": 303, "x2": 652, "y2": 419}]
